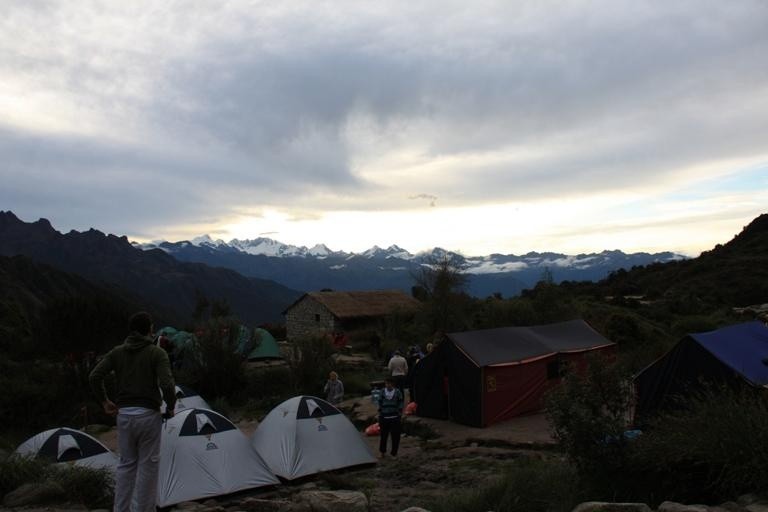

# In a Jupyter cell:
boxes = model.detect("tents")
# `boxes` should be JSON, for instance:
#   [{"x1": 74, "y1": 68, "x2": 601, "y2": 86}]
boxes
[
  {"x1": 158, "y1": 383, "x2": 213, "y2": 421},
  {"x1": 408, "y1": 319, "x2": 617, "y2": 428},
  {"x1": 632, "y1": 320, "x2": 768, "y2": 431},
  {"x1": 6, "y1": 427, "x2": 120, "y2": 482},
  {"x1": 251, "y1": 395, "x2": 379, "y2": 481},
  {"x1": 153, "y1": 327, "x2": 281, "y2": 380},
  {"x1": 156, "y1": 407, "x2": 281, "y2": 509}
]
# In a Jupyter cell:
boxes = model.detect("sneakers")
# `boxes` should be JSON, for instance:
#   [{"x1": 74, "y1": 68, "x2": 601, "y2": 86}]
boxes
[{"x1": 377, "y1": 452, "x2": 398, "y2": 460}]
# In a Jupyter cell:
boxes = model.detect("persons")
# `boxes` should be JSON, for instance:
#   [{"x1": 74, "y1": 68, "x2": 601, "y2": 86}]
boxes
[
  {"x1": 64, "y1": 348, "x2": 108, "y2": 431},
  {"x1": 322, "y1": 329, "x2": 446, "y2": 403},
  {"x1": 88, "y1": 311, "x2": 177, "y2": 512},
  {"x1": 157, "y1": 330, "x2": 191, "y2": 362},
  {"x1": 376, "y1": 378, "x2": 404, "y2": 461}
]
